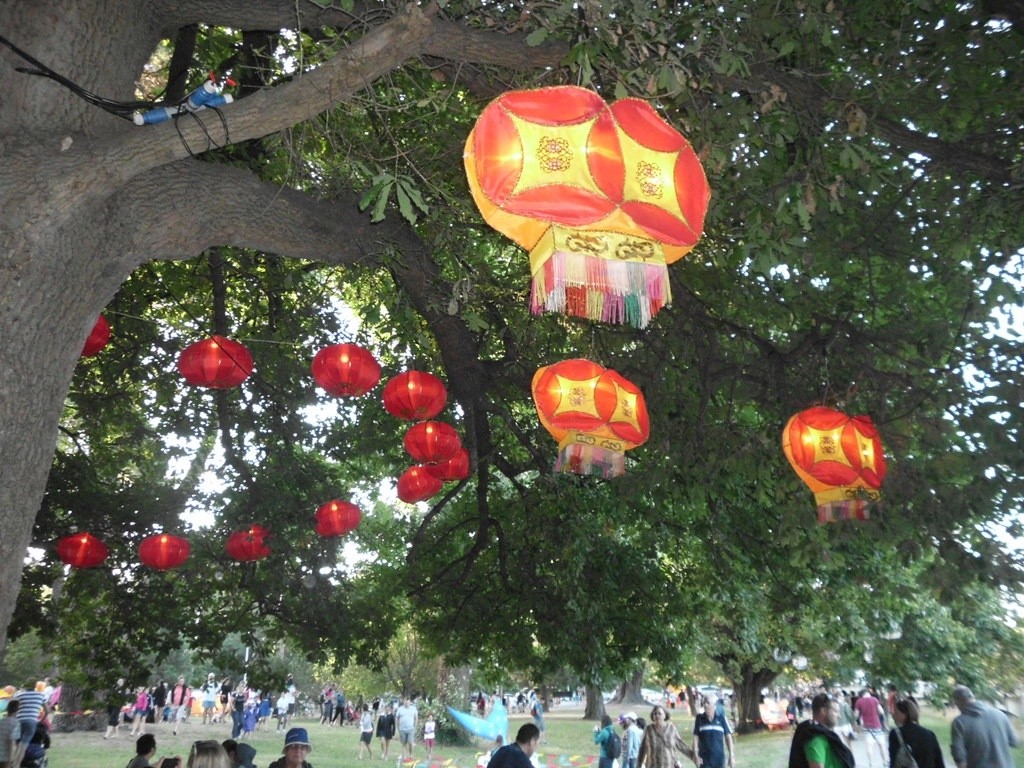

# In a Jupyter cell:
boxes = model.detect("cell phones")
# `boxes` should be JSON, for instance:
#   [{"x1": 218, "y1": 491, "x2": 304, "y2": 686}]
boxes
[{"x1": 160, "y1": 758, "x2": 179, "y2": 768}]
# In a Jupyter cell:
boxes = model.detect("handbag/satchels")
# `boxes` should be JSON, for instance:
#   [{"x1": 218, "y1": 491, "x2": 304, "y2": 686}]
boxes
[
  {"x1": 674, "y1": 760, "x2": 682, "y2": 768},
  {"x1": 531, "y1": 709, "x2": 536, "y2": 716},
  {"x1": 894, "y1": 744, "x2": 918, "y2": 768},
  {"x1": 787, "y1": 712, "x2": 795, "y2": 720}
]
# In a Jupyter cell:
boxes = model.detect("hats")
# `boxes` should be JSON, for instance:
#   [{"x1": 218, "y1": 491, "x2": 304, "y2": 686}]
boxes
[
  {"x1": 233, "y1": 743, "x2": 257, "y2": 768},
  {"x1": 282, "y1": 728, "x2": 312, "y2": 754},
  {"x1": 0, "y1": 685, "x2": 16, "y2": 698},
  {"x1": 623, "y1": 711, "x2": 638, "y2": 722},
  {"x1": 34, "y1": 681, "x2": 47, "y2": 691}
]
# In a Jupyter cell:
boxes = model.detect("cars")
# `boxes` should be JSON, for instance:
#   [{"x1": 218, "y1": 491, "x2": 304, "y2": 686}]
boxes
[
  {"x1": 905, "y1": 681, "x2": 935, "y2": 698},
  {"x1": 694, "y1": 685, "x2": 732, "y2": 699}
]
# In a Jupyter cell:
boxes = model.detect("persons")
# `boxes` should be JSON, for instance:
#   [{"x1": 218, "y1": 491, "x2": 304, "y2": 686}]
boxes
[
  {"x1": 104, "y1": 668, "x2": 297, "y2": 741},
  {"x1": 594, "y1": 687, "x2": 735, "y2": 768},
  {"x1": 319, "y1": 687, "x2": 346, "y2": 727},
  {"x1": 0, "y1": 678, "x2": 61, "y2": 768},
  {"x1": 355, "y1": 696, "x2": 435, "y2": 760},
  {"x1": 755, "y1": 683, "x2": 1020, "y2": 768},
  {"x1": 115, "y1": 728, "x2": 316, "y2": 768},
  {"x1": 486, "y1": 723, "x2": 541, "y2": 768},
  {"x1": 477, "y1": 688, "x2": 544, "y2": 756}
]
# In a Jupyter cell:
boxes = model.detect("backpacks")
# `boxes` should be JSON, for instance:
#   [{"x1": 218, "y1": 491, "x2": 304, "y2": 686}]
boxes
[{"x1": 606, "y1": 726, "x2": 622, "y2": 758}]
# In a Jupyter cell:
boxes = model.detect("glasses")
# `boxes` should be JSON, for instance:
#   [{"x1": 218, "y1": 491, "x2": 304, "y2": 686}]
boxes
[
  {"x1": 820, "y1": 692, "x2": 832, "y2": 706},
  {"x1": 285, "y1": 744, "x2": 309, "y2": 751}
]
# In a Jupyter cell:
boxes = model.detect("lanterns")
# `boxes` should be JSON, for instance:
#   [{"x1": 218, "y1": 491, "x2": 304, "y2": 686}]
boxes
[
  {"x1": 225, "y1": 523, "x2": 273, "y2": 561},
  {"x1": 382, "y1": 368, "x2": 447, "y2": 421},
  {"x1": 54, "y1": 531, "x2": 108, "y2": 569},
  {"x1": 313, "y1": 500, "x2": 360, "y2": 538},
  {"x1": 310, "y1": 342, "x2": 382, "y2": 398},
  {"x1": 531, "y1": 358, "x2": 650, "y2": 478},
  {"x1": 177, "y1": 334, "x2": 253, "y2": 393},
  {"x1": 397, "y1": 421, "x2": 469, "y2": 505},
  {"x1": 139, "y1": 532, "x2": 190, "y2": 572},
  {"x1": 781, "y1": 405, "x2": 886, "y2": 523},
  {"x1": 464, "y1": 83, "x2": 713, "y2": 329},
  {"x1": 83, "y1": 315, "x2": 111, "y2": 358}
]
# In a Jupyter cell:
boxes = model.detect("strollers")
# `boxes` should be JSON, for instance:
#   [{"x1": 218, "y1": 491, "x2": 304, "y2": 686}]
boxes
[{"x1": 14, "y1": 711, "x2": 52, "y2": 768}]
